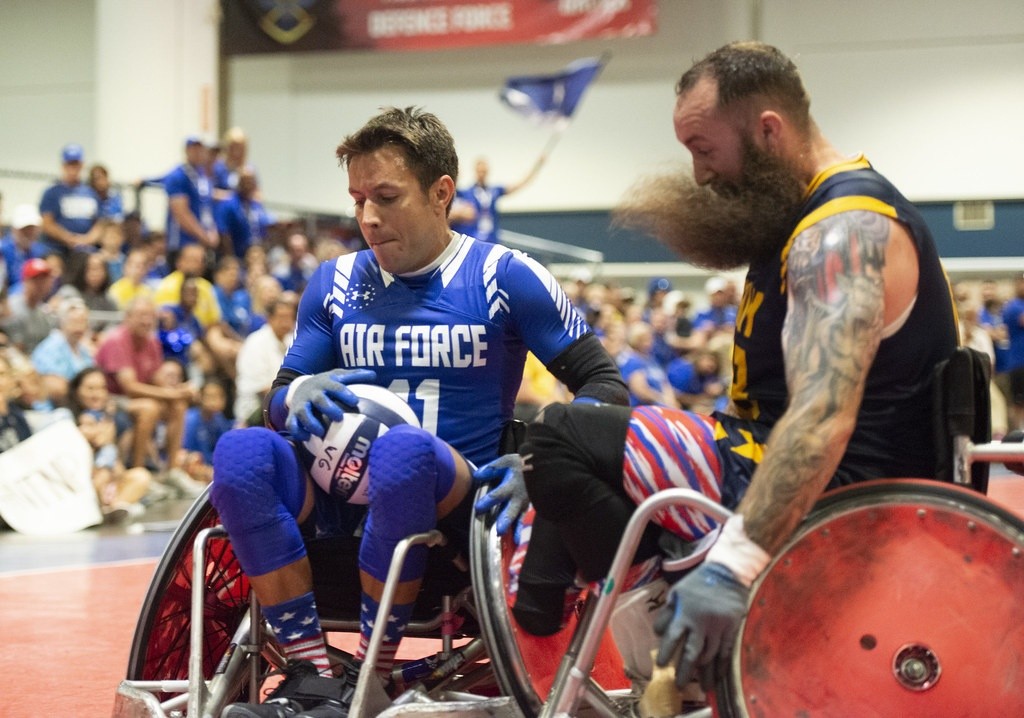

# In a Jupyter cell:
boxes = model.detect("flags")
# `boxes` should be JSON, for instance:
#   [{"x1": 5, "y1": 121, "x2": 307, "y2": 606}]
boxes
[{"x1": 498, "y1": 61, "x2": 600, "y2": 127}]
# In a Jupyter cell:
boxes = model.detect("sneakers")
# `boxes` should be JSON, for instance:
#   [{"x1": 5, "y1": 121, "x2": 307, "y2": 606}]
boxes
[
  {"x1": 220, "y1": 658, "x2": 341, "y2": 718},
  {"x1": 292, "y1": 663, "x2": 395, "y2": 718}
]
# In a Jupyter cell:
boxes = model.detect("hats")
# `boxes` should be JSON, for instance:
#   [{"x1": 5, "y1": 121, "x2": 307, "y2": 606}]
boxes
[
  {"x1": 649, "y1": 278, "x2": 671, "y2": 292},
  {"x1": 61, "y1": 143, "x2": 82, "y2": 163},
  {"x1": 20, "y1": 259, "x2": 53, "y2": 280},
  {"x1": 185, "y1": 136, "x2": 207, "y2": 147}
]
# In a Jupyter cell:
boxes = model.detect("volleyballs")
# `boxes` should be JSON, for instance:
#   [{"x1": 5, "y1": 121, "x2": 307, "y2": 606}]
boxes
[{"x1": 294, "y1": 381, "x2": 425, "y2": 508}]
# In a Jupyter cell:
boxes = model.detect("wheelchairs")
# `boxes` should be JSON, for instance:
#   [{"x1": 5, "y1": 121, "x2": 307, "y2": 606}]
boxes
[{"x1": 108, "y1": 347, "x2": 1023, "y2": 717}]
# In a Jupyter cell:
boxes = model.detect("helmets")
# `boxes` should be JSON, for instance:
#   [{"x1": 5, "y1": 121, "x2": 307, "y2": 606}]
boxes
[
  {"x1": 662, "y1": 293, "x2": 691, "y2": 316},
  {"x1": 704, "y1": 276, "x2": 731, "y2": 300}
]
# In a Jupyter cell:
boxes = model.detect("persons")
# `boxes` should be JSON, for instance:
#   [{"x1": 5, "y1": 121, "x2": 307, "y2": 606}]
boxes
[
  {"x1": 500, "y1": 40, "x2": 961, "y2": 718},
  {"x1": 446, "y1": 158, "x2": 542, "y2": 245},
  {"x1": 515, "y1": 268, "x2": 1024, "y2": 442},
  {"x1": 209, "y1": 105, "x2": 629, "y2": 718},
  {"x1": 0, "y1": 126, "x2": 349, "y2": 521}
]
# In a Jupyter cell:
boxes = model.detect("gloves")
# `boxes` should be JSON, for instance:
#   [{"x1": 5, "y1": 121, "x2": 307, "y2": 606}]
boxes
[
  {"x1": 471, "y1": 452, "x2": 533, "y2": 545},
  {"x1": 284, "y1": 369, "x2": 377, "y2": 442},
  {"x1": 653, "y1": 561, "x2": 752, "y2": 693}
]
